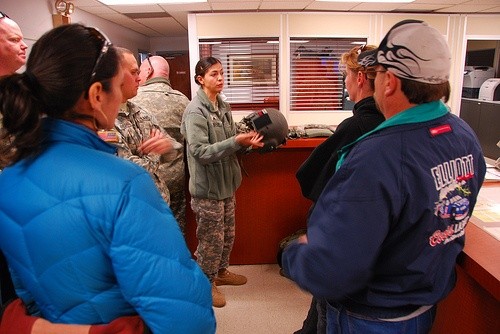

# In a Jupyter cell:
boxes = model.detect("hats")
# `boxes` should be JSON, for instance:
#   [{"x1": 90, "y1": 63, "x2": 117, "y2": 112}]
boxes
[{"x1": 357, "y1": 19, "x2": 451, "y2": 85}]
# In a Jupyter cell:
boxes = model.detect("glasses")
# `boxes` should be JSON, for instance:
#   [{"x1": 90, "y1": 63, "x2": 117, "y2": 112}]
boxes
[
  {"x1": 0, "y1": 11, "x2": 11, "y2": 19},
  {"x1": 365, "y1": 66, "x2": 386, "y2": 80},
  {"x1": 84, "y1": 25, "x2": 113, "y2": 100},
  {"x1": 146, "y1": 52, "x2": 155, "y2": 71}
]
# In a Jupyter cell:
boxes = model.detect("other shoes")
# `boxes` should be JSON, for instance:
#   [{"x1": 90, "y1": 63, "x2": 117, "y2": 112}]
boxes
[
  {"x1": 215, "y1": 268, "x2": 248, "y2": 287},
  {"x1": 211, "y1": 281, "x2": 227, "y2": 308}
]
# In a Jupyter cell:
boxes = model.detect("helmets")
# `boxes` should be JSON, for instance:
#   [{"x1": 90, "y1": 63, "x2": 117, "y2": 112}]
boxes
[{"x1": 242, "y1": 108, "x2": 288, "y2": 154}]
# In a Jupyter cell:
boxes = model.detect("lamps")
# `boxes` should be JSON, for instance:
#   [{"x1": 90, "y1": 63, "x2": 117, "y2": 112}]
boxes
[{"x1": 52, "y1": 0, "x2": 74, "y2": 28}]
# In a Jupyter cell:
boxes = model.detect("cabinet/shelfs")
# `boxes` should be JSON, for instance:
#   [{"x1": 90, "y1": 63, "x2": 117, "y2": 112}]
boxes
[{"x1": 459, "y1": 99, "x2": 500, "y2": 160}]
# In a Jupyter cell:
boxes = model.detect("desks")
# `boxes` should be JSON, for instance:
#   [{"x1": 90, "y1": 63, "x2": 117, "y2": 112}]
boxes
[
  {"x1": 183, "y1": 135, "x2": 330, "y2": 267},
  {"x1": 431, "y1": 179, "x2": 500, "y2": 334}
]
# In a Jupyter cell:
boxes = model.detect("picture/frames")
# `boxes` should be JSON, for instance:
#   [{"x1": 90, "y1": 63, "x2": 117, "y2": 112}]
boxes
[{"x1": 227, "y1": 53, "x2": 279, "y2": 88}]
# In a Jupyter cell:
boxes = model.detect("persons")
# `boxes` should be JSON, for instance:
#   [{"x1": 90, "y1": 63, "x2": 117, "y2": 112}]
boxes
[
  {"x1": 0, "y1": 24, "x2": 218, "y2": 334},
  {"x1": 96, "y1": 46, "x2": 183, "y2": 209},
  {"x1": 128, "y1": 53, "x2": 191, "y2": 237},
  {"x1": 181, "y1": 57, "x2": 263, "y2": 307},
  {"x1": 0, "y1": 11, "x2": 29, "y2": 170},
  {"x1": 281, "y1": 19, "x2": 486, "y2": 334},
  {"x1": 293, "y1": 45, "x2": 387, "y2": 334}
]
